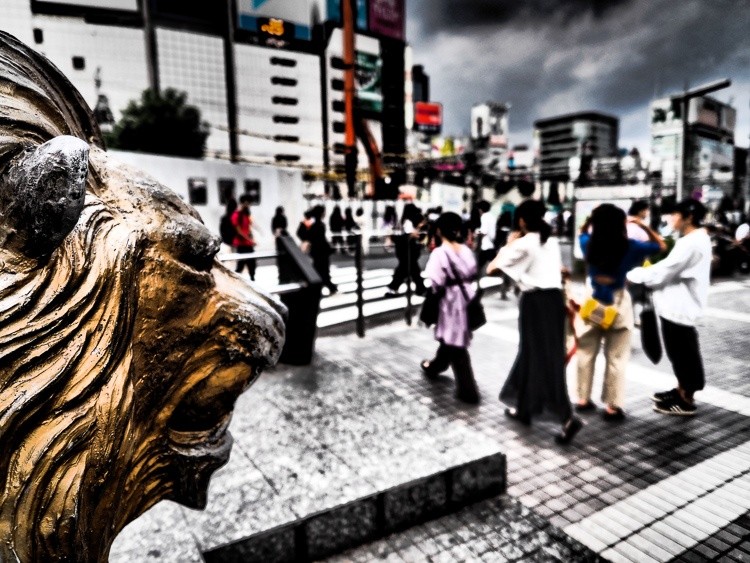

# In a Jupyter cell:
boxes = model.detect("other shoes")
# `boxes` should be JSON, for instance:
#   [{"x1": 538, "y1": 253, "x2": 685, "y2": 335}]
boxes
[
  {"x1": 604, "y1": 408, "x2": 625, "y2": 420},
  {"x1": 384, "y1": 292, "x2": 399, "y2": 298},
  {"x1": 421, "y1": 360, "x2": 441, "y2": 378},
  {"x1": 501, "y1": 295, "x2": 510, "y2": 301},
  {"x1": 554, "y1": 417, "x2": 583, "y2": 446},
  {"x1": 653, "y1": 398, "x2": 697, "y2": 415},
  {"x1": 454, "y1": 392, "x2": 479, "y2": 405},
  {"x1": 650, "y1": 388, "x2": 680, "y2": 403},
  {"x1": 514, "y1": 284, "x2": 521, "y2": 298},
  {"x1": 330, "y1": 284, "x2": 338, "y2": 296},
  {"x1": 575, "y1": 400, "x2": 599, "y2": 414},
  {"x1": 505, "y1": 408, "x2": 532, "y2": 427},
  {"x1": 416, "y1": 287, "x2": 428, "y2": 296}
]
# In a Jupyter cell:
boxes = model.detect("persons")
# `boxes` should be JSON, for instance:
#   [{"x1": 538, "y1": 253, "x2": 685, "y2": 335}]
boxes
[
  {"x1": 624, "y1": 194, "x2": 750, "y2": 244},
  {"x1": 419, "y1": 211, "x2": 481, "y2": 405},
  {"x1": 486, "y1": 200, "x2": 582, "y2": 445},
  {"x1": 461, "y1": 195, "x2": 575, "y2": 301},
  {"x1": 575, "y1": 202, "x2": 668, "y2": 423},
  {"x1": 625, "y1": 195, "x2": 712, "y2": 416},
  {"x1": 218, "y1": 181, "x2": 445, "y2": 297}
]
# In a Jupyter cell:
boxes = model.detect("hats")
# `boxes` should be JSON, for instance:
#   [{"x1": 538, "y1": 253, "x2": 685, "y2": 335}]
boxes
[{"x1": 676, "y1": 197, "x2": 708, "y2": 220}]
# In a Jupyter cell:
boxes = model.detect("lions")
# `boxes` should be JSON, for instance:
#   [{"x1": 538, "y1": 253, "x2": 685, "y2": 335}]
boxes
[{"x1": 1, "y1": 31, "x2": 289, "y2": 563}]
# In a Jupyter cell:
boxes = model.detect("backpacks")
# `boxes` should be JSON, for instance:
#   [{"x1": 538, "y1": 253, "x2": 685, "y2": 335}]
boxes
[
  {"x1": 493, "y1": 210, "x2": 514, "y2": 251},
  {"x1": 219, "y1": 198, "x2": 242, "y2": 245}
]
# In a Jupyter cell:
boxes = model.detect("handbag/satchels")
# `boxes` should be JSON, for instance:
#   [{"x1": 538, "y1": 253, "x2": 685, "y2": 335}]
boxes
[
  {"x1": 566, "y1": 298, "x2": 581, "y2": 336},
  {"x1": 579, "y1": 298, "x2": 618, "y2": 331},
  {"x1": 639, "y1": 284, "x2": 662, "y2": 364},
  {"x1": 466, "y1": 287, "x2": 487, "y2": 332}
]
